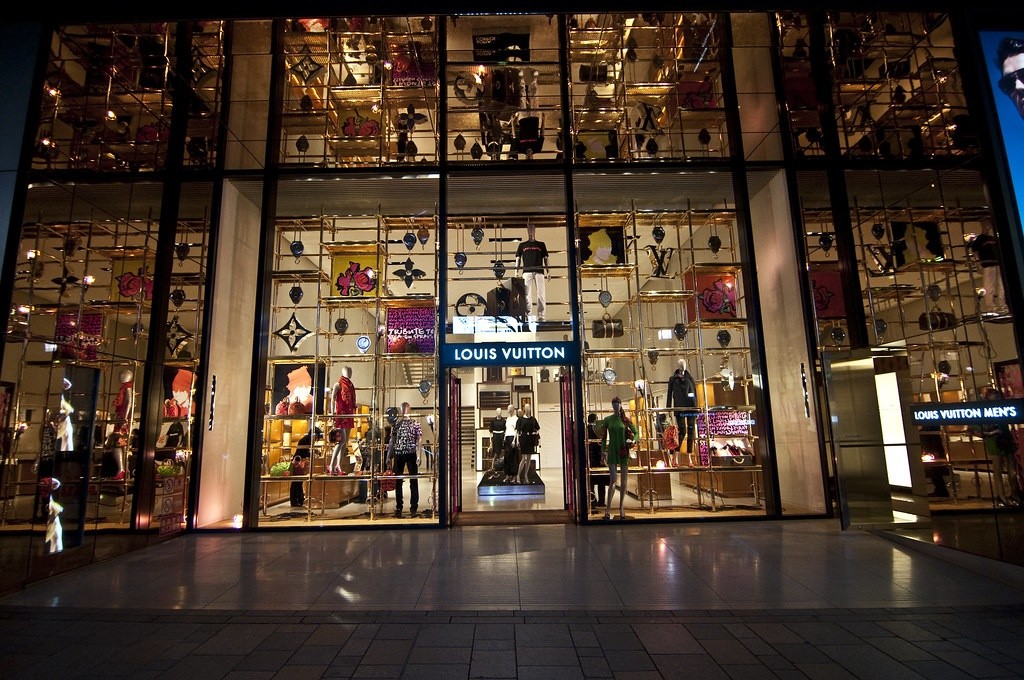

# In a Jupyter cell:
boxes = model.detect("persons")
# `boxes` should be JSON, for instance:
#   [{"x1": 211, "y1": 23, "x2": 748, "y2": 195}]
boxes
[
  {"x1": 163, "y1": 421, "x2": 184, "y2": 447},
  {"x1": 131, "y1": 429, "x2": 139, "y2": 455},
  {"x1": 540, "y1": 365, "x2": 550, "y2": 382},
  {"x1": 289, "y1": 427, "x2": 323, "y2": 507},
  {"x1": 104, "y1": 370, "x2": 133, "y2": 480},
  {"x1": 973, "y1": 217, "x2": 1013, "y2": 316},
  {"x1": 601, "y1": 397, "x2": 638, "y2": 519},
  {"x1": 919, "y1": 426, "x2": 950, "y2": 497},
  {"x1": 515, "y1": 224, "x2": 551, "y2": 321},
  {"x1": 348, "y1": 417, "x2": 392, "y2": 505},
  {"x1": 327, "y1": 365, "x2": 357, "y2": 476},
  {"x1": 587, "y1": 413, "x2": 607, "y2": 507},
  {"x1": 386, "y1": 402, "x2": 424, "y2": 518},
  {"x1": 666, "y1": 358, "x2": 698, "y2": 467},
  {"x1": 971, "y1": 389, "x2": 1024, "y2": 510},
  {"x1": 486, "y1": 403, "x2": 540, "y2": 484}
]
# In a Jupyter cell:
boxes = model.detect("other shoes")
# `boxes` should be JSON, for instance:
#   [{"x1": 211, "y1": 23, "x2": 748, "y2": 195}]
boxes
[
  {"x1": 411, "y1": 510, "x2": 416, "y2": 518},
  {"x1": 673, "y1": 463, "x2": 679, "y2": 467},
  {"x1": 395, "y1": 510, "x2": 402, "y2": 518},
  {"x1": 928, "y1": 491, "x2": 949, "y2": 497},
  {"x1": 996, "y1": 496, "x2": 1013, "y2": 509},
  {"x1": 112, "y1": 471, "x2": 125, "y2": 480},
  {"x1": 689, "y1": 463, "x2": 694, "y2": 467}
]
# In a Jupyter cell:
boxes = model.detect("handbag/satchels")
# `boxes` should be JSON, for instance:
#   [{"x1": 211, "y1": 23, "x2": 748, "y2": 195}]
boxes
[
  {"x1": 662, "y1": 415, "x2": 679, "y2": 450},
  {"x1": 382, "y1": 461, "x2": 396, "y2": 491},
  {"x1": 329, "y1": 419, "x2": 343, "y2": 443}
]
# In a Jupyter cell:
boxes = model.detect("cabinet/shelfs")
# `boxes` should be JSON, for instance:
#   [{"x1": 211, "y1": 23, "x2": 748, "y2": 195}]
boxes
[
  {"x1": 574, "y1": 207, "x2": 762, "y2": 514},
  {"x1": 768, "y1": 8, "x2": 982, "y2": 158},
  {"x1": 447, "y1": 61, "x2": 557, "y2": 162},
  {"x1": 0, "y1": 206, "x2": 210, "y2": 533},
  {"x1": 258, "y1": 384, "x2": 371, "y2": 508},
  {"x1": 475, "y1": 368, "x2": 541, "y2": 472},
  {"x1": 279, "y1": 15, "x2": 441, "y2": 169},
  {"x1": 258, "y1": 212, "x2": 439, "y2": 520},
  {"x1": 850, "y1": 205, "x2": 1023, "y2": 511},
  {"x1": 31, "y1": 21, "x2": 226, "y2": 170},
  {"x1": 677, "y1": 375, "x2": 764, "y2": 498},
  {"x1": 563, "y1": 11, "x2": 731, "y2": 162},
  {"x1": 444, "y1": 250, "x2": 572, "y2": 335}
]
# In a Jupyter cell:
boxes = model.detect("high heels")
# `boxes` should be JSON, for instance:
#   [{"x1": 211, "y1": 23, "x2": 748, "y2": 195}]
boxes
[
  {"x1": 327, "y1": 465, "x2": 333, "y2": 475},
  {"x1": 335, "y1": 466, "x2": 346, "y2": 475}
]
